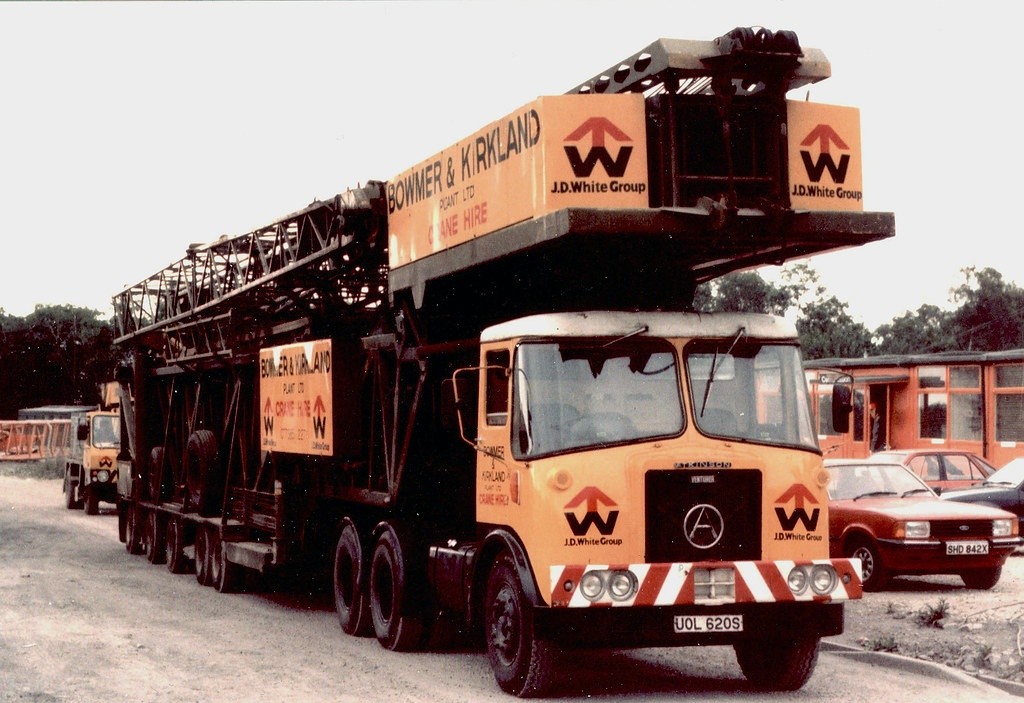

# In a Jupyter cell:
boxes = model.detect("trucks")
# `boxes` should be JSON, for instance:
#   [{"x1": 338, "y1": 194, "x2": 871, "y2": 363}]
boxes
[{"x1": 62, "y1": 412, "x2": 122, "y2": 514}]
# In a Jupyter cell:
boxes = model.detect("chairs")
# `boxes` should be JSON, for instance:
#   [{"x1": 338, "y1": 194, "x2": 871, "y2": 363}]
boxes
[
  {"x1": 529, "y1": 404, "x2": 737, "y2": 456},
  {"x1": 836, "y1": 476, "x2": 859, "y2": 499},
  {"x1": 923, "y1": 460, "x2": 940, "y2": 480}
]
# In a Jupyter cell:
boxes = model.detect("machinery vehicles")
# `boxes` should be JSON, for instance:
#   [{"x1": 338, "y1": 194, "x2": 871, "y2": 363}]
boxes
[{"x1": 62, "y1": 27, "x2": 898, "y2": 697}]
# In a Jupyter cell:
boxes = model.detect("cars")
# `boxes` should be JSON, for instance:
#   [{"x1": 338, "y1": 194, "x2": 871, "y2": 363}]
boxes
[
  {"x1": 869, "y1": 449, "x2": 1024, "y2": 535},
  {"x1": 820, "y1": 459, "x2": 1024, "y2": 592}
]
